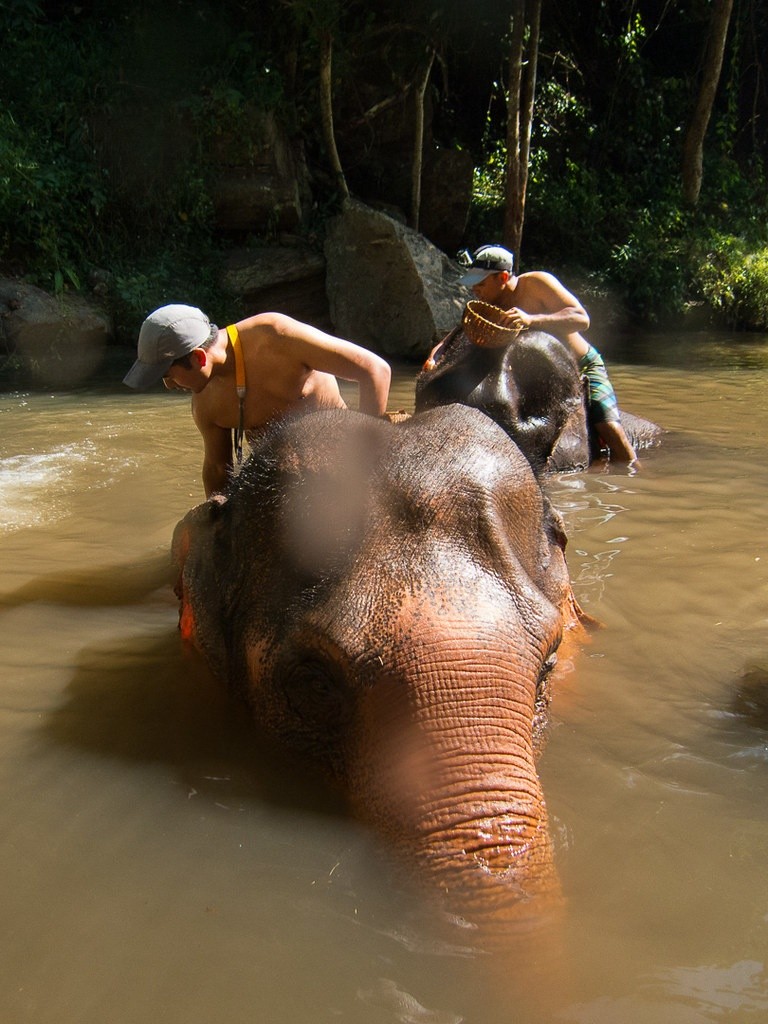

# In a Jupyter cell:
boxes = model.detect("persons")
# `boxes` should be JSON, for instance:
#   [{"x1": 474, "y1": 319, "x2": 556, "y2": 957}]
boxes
[
  {"x1": 121, "y1": 302, "x2": 412, "y2": 501},
  {"x1": 456, "y1": 248, "x2": 636, "y2": 460}
]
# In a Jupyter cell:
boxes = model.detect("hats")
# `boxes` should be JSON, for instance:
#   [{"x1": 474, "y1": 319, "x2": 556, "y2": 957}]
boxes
[
  {"x1": 122, "y1": 304, "x2": 210, "y2": 390},
  {"x1": 459, "y1": 245, "x2": 513, "y2": 286}
]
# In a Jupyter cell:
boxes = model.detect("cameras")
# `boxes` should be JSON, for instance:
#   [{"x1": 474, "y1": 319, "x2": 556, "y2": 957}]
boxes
[{"x1": 456, "y1": 249, "x2": 473, "y2": 265}]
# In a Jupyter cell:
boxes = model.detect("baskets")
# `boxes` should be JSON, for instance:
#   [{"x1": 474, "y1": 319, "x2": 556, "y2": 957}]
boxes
[{"x1": 461, "y1": 299, "x2": 522, "y2": 350}]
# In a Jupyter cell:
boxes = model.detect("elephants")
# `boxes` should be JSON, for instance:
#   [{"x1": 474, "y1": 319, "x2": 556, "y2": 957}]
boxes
[
  {"x1": 416, "y1": 332, "x2": 668, "y2": 476},
  {"x1": 170, "y1": 402, "x2": 605, "y2": 975}
]
